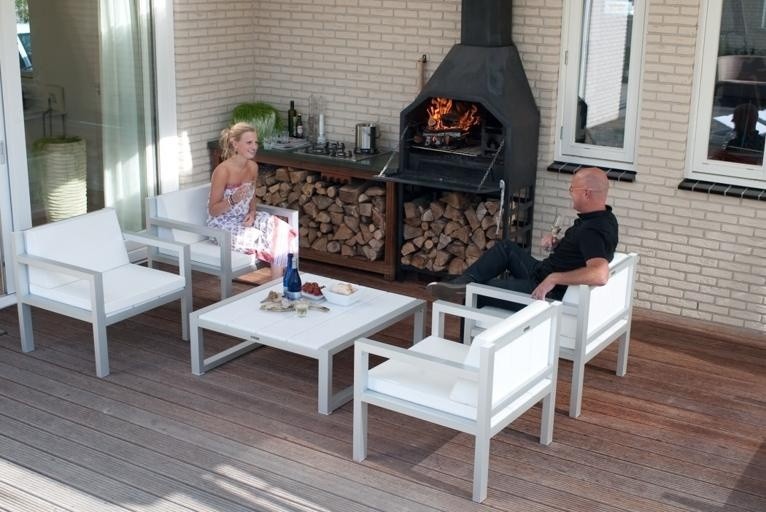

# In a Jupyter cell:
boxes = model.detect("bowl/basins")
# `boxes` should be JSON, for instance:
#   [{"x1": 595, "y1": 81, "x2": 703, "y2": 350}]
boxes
[{"x1": 321, "y1": 281, "x2": 366, "y2": 306}]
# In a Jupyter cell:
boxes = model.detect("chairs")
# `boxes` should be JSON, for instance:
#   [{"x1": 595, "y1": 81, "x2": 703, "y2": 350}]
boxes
[
  {"x1": 144, "y1": 182, "x2": 299, "y2": 300},
  {"x1": 9, "y1": 207, "x2": 194, "y2": 379},
  {"x1": 463, "y1": 252, "x2": 639, "y2": 419},
  {"x1": 352, "y1": 298, "x2": 564, "y2": 504},
  {"x1": 715, "y1": 55, "x2": 766, "y2": 108}
]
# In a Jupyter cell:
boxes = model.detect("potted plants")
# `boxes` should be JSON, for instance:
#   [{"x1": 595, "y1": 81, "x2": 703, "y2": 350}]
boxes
[
  {"x1": 232, "y1": 101, "x2": 285, "y2": 147},
  {"x1": 31, "y1": 137, "x2": 88, "y2": 224}
]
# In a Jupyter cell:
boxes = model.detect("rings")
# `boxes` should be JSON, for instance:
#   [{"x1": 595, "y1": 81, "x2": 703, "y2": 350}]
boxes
[{"x1": 538, "y1": 294, "x2": 541, "y2": 296}]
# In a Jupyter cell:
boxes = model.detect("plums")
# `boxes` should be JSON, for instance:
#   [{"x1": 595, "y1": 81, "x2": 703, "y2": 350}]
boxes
[{"x1": 301, "y1": 282, "x2": 321, "y2": 296}]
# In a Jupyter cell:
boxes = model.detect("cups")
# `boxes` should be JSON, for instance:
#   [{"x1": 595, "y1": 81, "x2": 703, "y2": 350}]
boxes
[{"x1": 263, "y1": 128, "x2": 291, "y2": 151}]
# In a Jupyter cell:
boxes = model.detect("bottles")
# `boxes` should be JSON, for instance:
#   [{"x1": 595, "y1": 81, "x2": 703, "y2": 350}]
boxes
[
  {"x1": 282, "y1": 253, "x2": 294, "y2": 297},
  {"x1": 287, "y1": 258, "x2": 302, "y2": 301},
  {"x1": 287, "y1": 99, "x2": 303, "y2": 137}
]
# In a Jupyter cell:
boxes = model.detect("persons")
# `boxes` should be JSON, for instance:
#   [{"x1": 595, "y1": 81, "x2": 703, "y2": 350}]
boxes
[
  {"x1": 204, "y1": 122, "x2": 296, "y2": 280},
  {"x1": 726, "y1": 101, "x2": 764, "y2": 155},
  {"x1": 426, "y1": 167, "x2": 617, "y2": 344}
]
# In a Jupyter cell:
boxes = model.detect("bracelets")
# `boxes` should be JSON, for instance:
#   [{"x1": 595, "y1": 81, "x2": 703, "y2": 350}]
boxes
[{"x1": 226, "y1": 193, "x2": 236, "y2": 207}]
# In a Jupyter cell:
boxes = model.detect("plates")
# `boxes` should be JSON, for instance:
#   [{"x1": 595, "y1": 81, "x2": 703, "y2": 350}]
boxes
[{"x1": 296, "y1": 285, "x2": 327, "y2": 301}]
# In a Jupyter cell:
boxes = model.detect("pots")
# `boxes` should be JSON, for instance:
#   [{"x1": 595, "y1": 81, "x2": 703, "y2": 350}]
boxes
[{"x1": 356, "y1": 122, "x2": 379, "y2": 153}]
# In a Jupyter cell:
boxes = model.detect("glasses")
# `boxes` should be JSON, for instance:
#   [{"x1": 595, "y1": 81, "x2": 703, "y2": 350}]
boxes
[{"x1": 569, "y1": 185, "x2": 586, "y2": 193}]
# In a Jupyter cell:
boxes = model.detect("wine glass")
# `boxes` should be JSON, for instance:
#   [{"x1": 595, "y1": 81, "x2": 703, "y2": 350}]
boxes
[{"x1": 244, "y1": 174, "x2": 254, "y2": 199}]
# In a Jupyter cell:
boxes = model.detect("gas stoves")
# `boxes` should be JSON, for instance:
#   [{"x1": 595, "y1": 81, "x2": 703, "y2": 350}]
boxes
[{"x1": 292, "y1": 142, "x2": 390, "y2": 162}]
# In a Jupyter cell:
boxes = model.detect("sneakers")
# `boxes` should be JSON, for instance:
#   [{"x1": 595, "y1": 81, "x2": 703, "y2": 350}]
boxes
[{"x1": 425, "y1": 276, "x2": 465, "y2": 301}]
